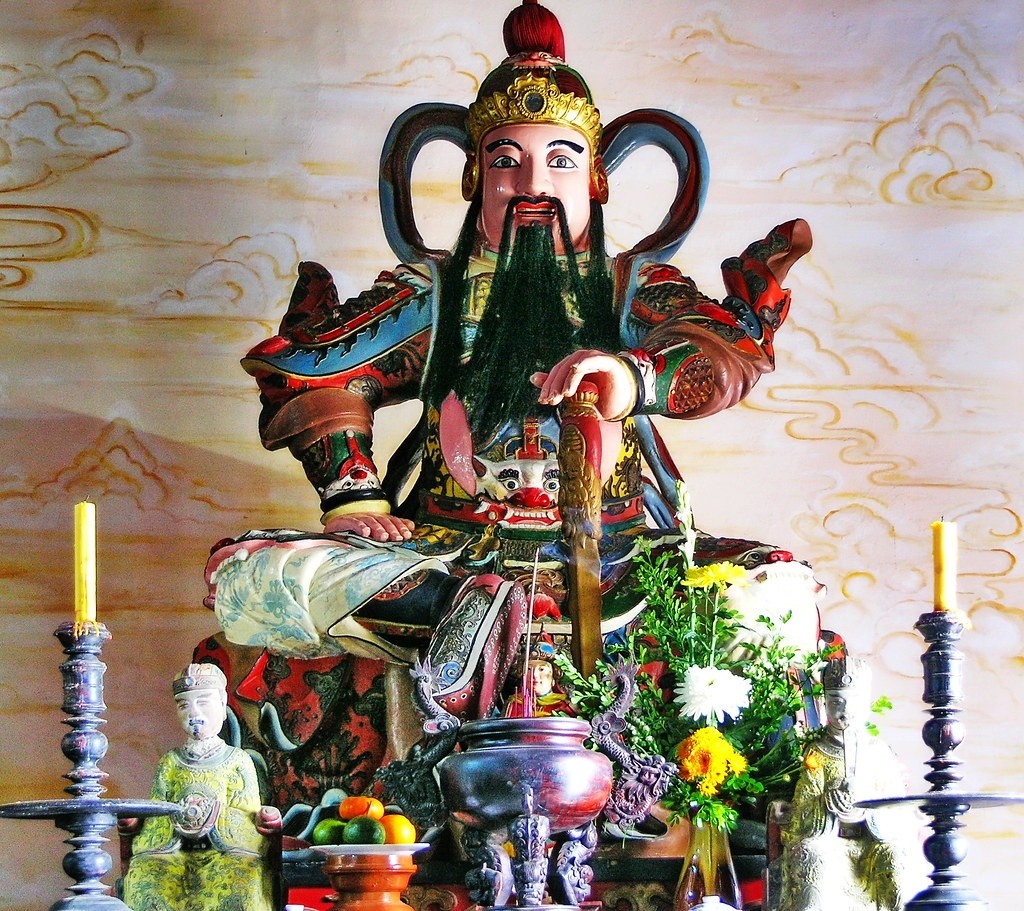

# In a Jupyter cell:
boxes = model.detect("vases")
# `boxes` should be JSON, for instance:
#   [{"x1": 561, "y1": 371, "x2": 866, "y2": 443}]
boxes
[{"x1": 669, "y1": 805, "x2": 742, "y2": 911}]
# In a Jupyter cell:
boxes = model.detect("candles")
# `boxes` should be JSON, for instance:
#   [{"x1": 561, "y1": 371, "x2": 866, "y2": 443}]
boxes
[
  {"x1": 75, "y1": 492, "x2": 97, "y2": 621},
  {"x1": 931, "y1": 517, "x2": 962, "y2": 610}
]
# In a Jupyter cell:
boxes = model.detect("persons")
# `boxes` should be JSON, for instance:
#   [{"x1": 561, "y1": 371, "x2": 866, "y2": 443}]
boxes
[
  {"x1": 124, "y1": 663, "x2": 272, "y2": 911},
  {"x1": 778, "y1": 661, "x2": 905, "y2": 911},
  {"x1": 503, "y1": 633, "x2": 576, "y2": 719},
  {"x1": 204, "y1": 0, "x2": 821, "y2": 726}
]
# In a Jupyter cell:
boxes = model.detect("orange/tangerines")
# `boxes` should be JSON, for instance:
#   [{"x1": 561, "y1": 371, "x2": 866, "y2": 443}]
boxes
[{"x1": 313, "y1": 796, "x2": 417, "y2": 844}]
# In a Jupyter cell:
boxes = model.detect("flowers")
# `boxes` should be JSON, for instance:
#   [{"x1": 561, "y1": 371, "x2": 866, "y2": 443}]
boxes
[{"x1": 549, "y1": 479, "x2": 838, "y2": 832}]
